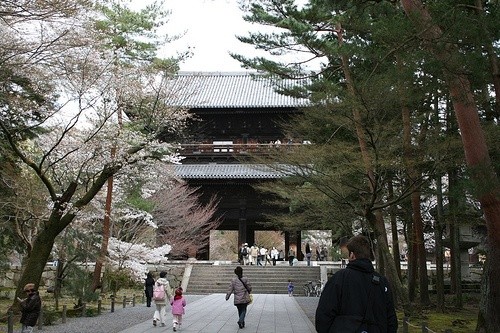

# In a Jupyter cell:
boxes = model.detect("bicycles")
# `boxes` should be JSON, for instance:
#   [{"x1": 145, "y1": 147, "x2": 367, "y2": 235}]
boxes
[
  {"x1": 303, "y1": 280, "x2": 316, "y2": 297},
  {"x1": 314, "y1": 280, "x2": 322, "y2": 298}
]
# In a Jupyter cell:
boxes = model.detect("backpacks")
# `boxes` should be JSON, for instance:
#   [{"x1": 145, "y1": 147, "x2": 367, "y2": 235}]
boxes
[
  {"x1": 152, "y1": 280, "x2": 166, "y2": 301},
  {"x1": 242, "y1": 248, "x2": 247, "y2": 256}
]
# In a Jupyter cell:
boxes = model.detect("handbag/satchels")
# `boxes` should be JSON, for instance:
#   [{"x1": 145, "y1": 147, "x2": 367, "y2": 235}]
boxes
[
  {"x1": 249, "y1": 292, "x2": 253, "y2": 303},
  {"x1": 292, "y1": 258, "x2": 298, "y2": 264}
]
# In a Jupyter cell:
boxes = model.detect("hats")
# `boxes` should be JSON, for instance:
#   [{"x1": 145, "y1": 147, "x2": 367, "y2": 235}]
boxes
[
  {"x1": 175, "y1": 287, "x2": 183, "y2": 296},
  {"x1": 235, "y1": 266, "x2": 242, "y2": 274},
  {"x1": 245, "y1": 243, "x2": 248, "y2": 246}
]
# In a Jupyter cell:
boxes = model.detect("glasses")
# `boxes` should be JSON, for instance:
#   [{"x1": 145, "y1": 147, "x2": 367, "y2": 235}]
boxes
[{"x1": 23, "y1": 290, "x2": 30, "y2": 293}]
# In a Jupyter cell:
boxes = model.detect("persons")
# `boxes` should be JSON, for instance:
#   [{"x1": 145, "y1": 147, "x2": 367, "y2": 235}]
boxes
[
  {"x1": 315, "y1": 235, "x2": 399, "y2": 333},
  {"x1": 170, "y1": 287, "x2": 187, "y2": 332},
  {"x1": 237, "y1": 243, "x2": 328, "y2": 267},
  {"x1": 145, "y1": 272, "x2": 155, "y2": 307},
  {"x1": 225, "y1": 266, "x2": 253, "y2": 329},
  {"x1": 152, "y1": 271, "x2": 172, "y2": 327},
  {"x1": 288, "y1": 280, "x2": 296, "y2": 297}
]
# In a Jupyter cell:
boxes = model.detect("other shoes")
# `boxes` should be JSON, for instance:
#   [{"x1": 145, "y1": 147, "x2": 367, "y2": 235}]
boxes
[
  {"x1": 237, "y1": 320, "x2": 245, "y2": 329},
  {"x1": 152, "y1": 319, "x2": 157, "y2": 327},
  {"x1": 172, "y1": 325, "x2": 177, "y2": 332},
  {"x1": 161, "y1": 322, "x2": 166, "y2": 327}
]
